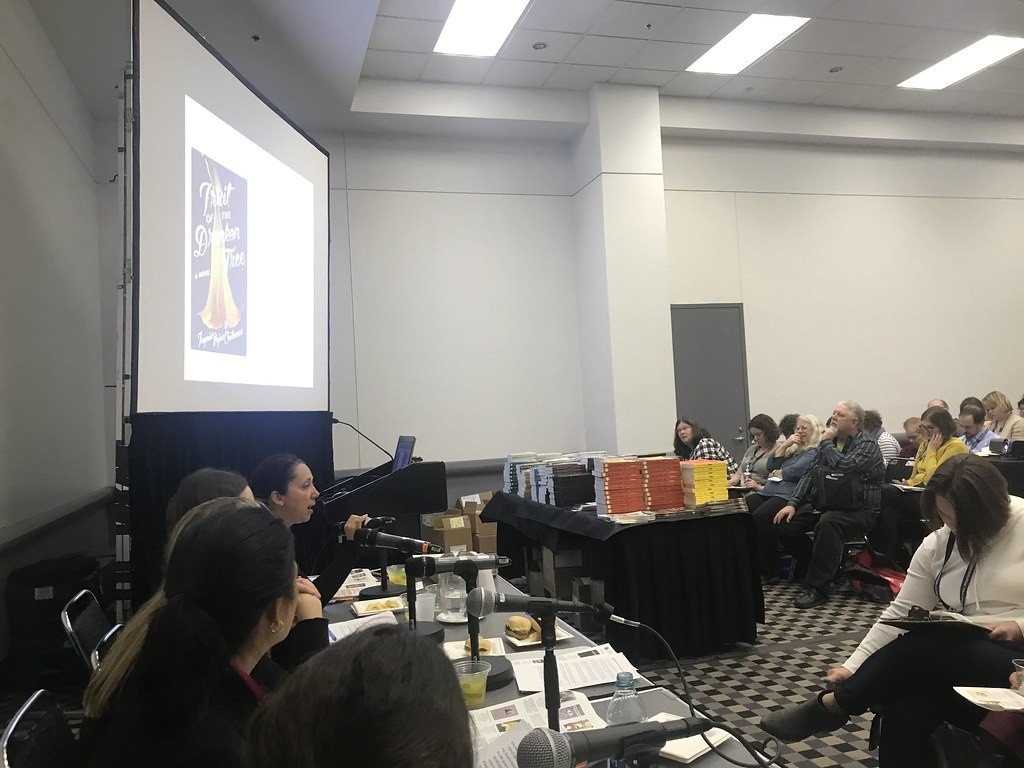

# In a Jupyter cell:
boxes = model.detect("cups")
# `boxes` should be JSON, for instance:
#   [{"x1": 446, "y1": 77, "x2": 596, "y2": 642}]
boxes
[
  {"x1": 457, "y1": 544, "x2": 466, "y2": 551},
  {"x1": 399, "y1": 593, "x2": 409, "y2": 621},
  {"x1": 1012, "y1": 659, "x2": 1024, "y2": 697},
  {"x1": 425, "y1": 584, "x2": 442, "y2": 612},
  {"x1": 452, "y1": 661, "x2": 491, "y2": 710},
  {"x1": 450, "y1": 546, "x2": 460, "y2": 557},
  {"x1": 415, "y1": 594, "x2": 435, "y2": 621},
  {"x1": 386, "y1": 564, "x2": 407, "y2": 586}
]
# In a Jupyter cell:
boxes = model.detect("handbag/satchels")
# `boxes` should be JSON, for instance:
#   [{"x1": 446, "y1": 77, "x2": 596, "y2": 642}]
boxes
[{"x1": 807, "y1": 435, "x2": 861, "y2": 512}]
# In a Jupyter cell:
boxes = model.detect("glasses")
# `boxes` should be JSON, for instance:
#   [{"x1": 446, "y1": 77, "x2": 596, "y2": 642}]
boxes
[
  {"x1": 1016, "y1": 406, "x2": 1023, "y2": 411},
  {"x1": 919, "y1": 424, "x2": 940, "y2": 432},
  {"x1": 676, "y1": 425, "x2": 691, "y2": 433},
  {"x1": 794, "y1": 425, "x2": 814, "y2": 432}
]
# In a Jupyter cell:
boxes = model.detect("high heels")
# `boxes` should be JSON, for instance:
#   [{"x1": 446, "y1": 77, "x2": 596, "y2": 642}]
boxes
[{"x1": 761, "y1": 691, "x2": 852, "y2": 744}]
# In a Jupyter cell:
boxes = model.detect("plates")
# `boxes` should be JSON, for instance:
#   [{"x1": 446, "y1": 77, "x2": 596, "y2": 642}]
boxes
[
  {"x1": 350, "y1": 597, "x2": 405, "y2": 616},
  {"x1": 327, "y1": 611, "x2": 398, "y2": 646},
  {"x1": 504, "y1": 624, "x2": 574, "y2": 647},
  {"x1": 443, "y1": 638, "x2": 506, "y2": 661},
  {"x1": 436, "y1": 613, "x2": 484, "y2": 623}
]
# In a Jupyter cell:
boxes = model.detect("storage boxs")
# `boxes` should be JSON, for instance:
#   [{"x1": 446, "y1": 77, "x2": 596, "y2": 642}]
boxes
[
  {"x1": 463, "y1": 500, "x2": 497, "y2": 536},
  {"x1": 455, "y1": 491, "x2": 493, "y2": 511},
  {"x1": 541, "y1": 544, "x2": 582, "y2": 596},
  {"x1": 557, "y1": 595, "x2": 582, "y2": 631},
  {"x1": 433, "y1": 515, "x2": 473, "y2": 553},
  {"x1": 574, "y1": 612, "x2": 603, "y2": 633},
  {"x1": 473, "y1": 533, "x2": 496, "y2": 553},
  {"x1": 572, "y1": 576, "x2": 606, "y2": 602},
  {"x1": 422, "y1": 508, "x2": 462, "y2": 542},
  {"x1": 529, "y1": 560, "x2": 545, "y2": 597}
]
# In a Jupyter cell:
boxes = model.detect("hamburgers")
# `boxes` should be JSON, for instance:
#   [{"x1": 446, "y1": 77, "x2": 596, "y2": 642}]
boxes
[
  {"x1": 464, "y1": 635, "x2": 492, "y2": 656},
  {"x1": 529, "y1": 617, "x2": 541, "y2": 641},
  {"x1": 504, "y1": 616, "x2": 532, "y2": 639}
]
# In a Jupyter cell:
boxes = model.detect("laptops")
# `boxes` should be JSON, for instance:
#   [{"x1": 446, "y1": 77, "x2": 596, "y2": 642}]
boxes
[{"x1": 392, "y1": 436, "x2": 416, "y2": 471}]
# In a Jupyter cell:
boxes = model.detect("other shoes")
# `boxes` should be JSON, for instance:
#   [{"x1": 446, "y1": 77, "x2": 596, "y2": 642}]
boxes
[
  {"x1": 900, "y1": 539, "x2": 913, "y2": 571},
  {"x1": 760, "y1": 570, "x2": 780, "y2": 585}
]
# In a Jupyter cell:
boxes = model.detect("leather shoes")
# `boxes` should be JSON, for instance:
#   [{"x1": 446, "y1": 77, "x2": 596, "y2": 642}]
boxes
[{"x1": 794, "y1": 588, "x2": 829, "y2": 607}]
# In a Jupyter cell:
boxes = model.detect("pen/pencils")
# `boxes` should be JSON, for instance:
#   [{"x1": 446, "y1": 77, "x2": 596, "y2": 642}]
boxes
[
  {"x1": 327, "y1": 627, "x2": 336, "y2": 641},
  {"x1": 327, "y1": 597, "x2": 354, "y2": 604}
]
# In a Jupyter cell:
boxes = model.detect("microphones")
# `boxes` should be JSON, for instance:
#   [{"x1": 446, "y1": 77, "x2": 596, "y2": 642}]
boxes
[
  {"x1": 516, "y1": 717, "x2": 714, "y2": 768},
  {"x1": 332, "y1": 517, "x2": 593, "y2": 618},
  {"x1": 328, "y1": 417, "x2": 393, "y2": 462}
]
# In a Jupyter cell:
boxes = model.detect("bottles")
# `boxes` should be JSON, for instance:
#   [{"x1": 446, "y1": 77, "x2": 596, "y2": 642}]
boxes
[{"x1": 605, "y1": 672, "x2": 648, "y2": 768}]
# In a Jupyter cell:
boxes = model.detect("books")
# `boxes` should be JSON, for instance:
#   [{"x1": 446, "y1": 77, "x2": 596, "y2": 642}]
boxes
[{"x1": 503, "y1": 450, "x2": 748, "y2": 524}]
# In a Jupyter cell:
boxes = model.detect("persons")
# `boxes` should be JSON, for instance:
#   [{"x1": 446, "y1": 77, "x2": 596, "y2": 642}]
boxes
[
  {"x1": 166, "y1": 468, "x2": 331, "y2": 666},
  {"x1": 728, "y1": 391, "x2": 1024, "y2": 609},
  {"x1": 673, "y1": 416, "x2": 741, "y2": 487},
  {"x1": 252, "y1": 453, "x2": 369, "y2": 610},
  {"x1": 251, "y1": 623, "x2": 474, "y2": 768},
  {"x1": 760, "y1": 452, "x2": 1024, "y2": 768},
  {"x1": 72, "y1": 498, "x2": 299, "y2": 765}
]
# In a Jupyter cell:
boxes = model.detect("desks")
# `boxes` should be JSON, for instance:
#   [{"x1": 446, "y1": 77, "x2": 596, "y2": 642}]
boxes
[
  {"x1": 479, "y1": 490, "x2": 766, "y2": 662},
  {"x1": 590, "y1": 686, "x2": 781, "y2": 768},
  {"x1": 323, "y1": 569, "x2": 657, "y2": 708}
]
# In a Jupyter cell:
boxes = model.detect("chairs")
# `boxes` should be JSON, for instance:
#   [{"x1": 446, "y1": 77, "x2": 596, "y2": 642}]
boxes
[
  {"x1": 0, "y1": 688, "x2": 76, "y2": 768},
  {"x1": 60, "y1": 590, "x2": 112, "y2": 674},
  {"x1": 788, "y1": 531, "x2": 871, "y2": 596},
  {"x1": 886, "y1": 457, "x2": 915, "y2": 483}
]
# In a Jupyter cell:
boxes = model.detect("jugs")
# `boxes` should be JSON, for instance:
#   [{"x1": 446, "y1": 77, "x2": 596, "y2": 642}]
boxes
[{"x1": 437, "y1": 557, "x2": 479, "y2": 620}]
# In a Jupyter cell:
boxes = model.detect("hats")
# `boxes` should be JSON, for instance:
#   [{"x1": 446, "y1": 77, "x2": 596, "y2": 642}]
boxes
[{"x1": 168, "y1": 468, "x2": 246, "y2": 533}]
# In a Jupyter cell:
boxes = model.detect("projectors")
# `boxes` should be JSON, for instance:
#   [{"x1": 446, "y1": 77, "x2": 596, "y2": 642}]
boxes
[{"x1": 989, "y1": 437, "x2": 1024, "y2": 458}]
